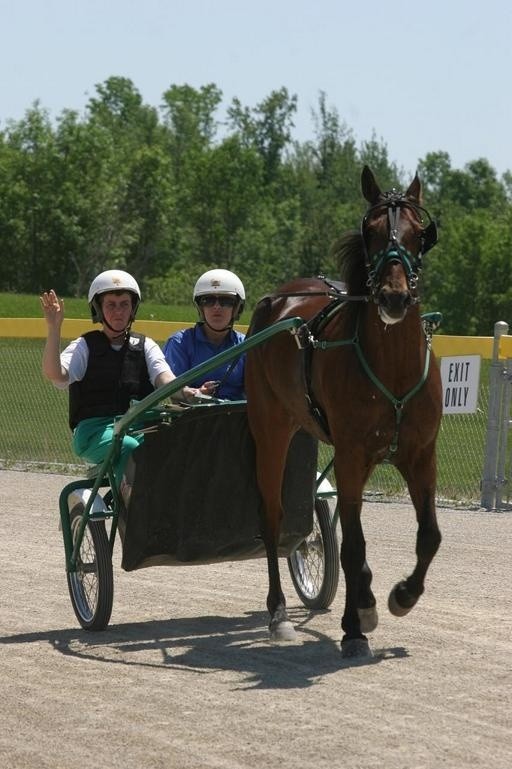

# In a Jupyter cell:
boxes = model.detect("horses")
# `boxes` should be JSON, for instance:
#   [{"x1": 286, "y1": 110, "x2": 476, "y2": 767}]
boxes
[{"x1": 241, "y1": 164, "x2": 445, "y2": 665}]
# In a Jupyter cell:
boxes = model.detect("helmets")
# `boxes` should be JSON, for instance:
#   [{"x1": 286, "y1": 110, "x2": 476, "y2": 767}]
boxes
[
  {"x1": 88, "y1": 269, "x2": 141, "y2": 303},
  {"x1": 193, "y1": 269, "x2": 246, "y2": 303}
]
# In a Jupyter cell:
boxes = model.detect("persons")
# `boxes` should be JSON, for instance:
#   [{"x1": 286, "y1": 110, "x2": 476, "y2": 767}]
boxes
[
  {"x1": 161, "y1": 269, "x2": 247, "y2": 400},
  {"x1": 39, "y1": 269, "x2": 202, "y2": 496}
]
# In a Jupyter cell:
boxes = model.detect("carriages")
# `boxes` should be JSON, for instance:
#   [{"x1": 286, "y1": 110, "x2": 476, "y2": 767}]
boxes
[{"x1": 57, "y1": 166, "x2": 443, "y2": 662}]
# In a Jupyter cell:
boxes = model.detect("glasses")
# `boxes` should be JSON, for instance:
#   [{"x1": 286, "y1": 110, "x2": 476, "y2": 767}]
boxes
[{"x1": 196, "y1": 295, "x2": 237, "y2": 307}]
